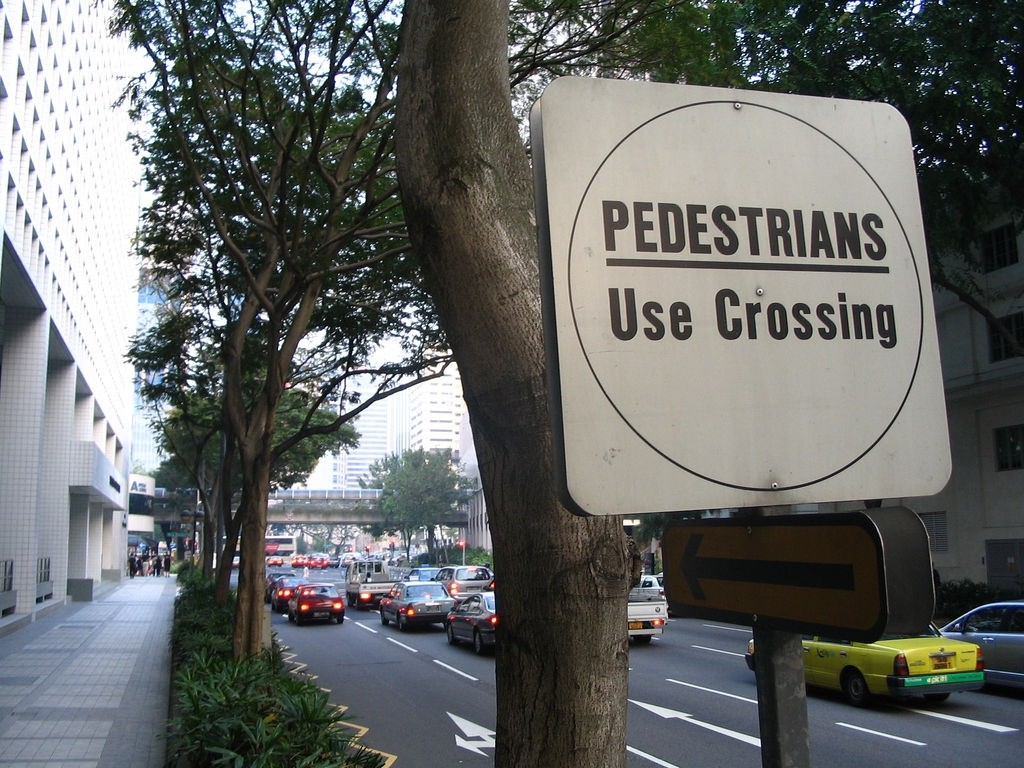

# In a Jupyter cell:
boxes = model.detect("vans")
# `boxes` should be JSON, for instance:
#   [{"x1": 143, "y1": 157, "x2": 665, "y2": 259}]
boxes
[{"x1": 431, "y1": 565, "x2": 495, "y2": 603}]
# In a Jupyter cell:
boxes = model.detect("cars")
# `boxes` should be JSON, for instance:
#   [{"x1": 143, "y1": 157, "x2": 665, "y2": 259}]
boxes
[
  {"x1": 231, "y1": 556, "x2": 241, "y2": 567},
  {"x1": 936, "y1": 599, "x2": 1024, "y2": 690},
  {"x1": 267, "y1": 555, "x2": 283, "y2": 566},
  {"x1": 291, "y1": 552, "x2": 413, "y2": 569},
  {"x1": 444, "y1": 592, "x2": 497, "y2": 654},
  {"x1": 264, "y1": 573, "x2": 345, "y2": 624},
  {"x1": 639, "y1": 575, "x2": 666, "y2": 600},
  {"x1": 744, "y1": 619, "x2": 986, "y2": 707},
  {"x1": 402, "y1": 567, "x2": 441, "y2": 581},
  {"x1": 378, "y1": 581, "x2": 459, "y2": 632}
]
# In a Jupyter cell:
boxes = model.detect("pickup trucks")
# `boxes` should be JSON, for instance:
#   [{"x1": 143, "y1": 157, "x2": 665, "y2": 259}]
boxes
[
  {"x1": 343, "y1": 559, "x2": 403, "y2": 609},
  {"x1": 627, "y1": 588, "x2": 669, "y2": 644}
]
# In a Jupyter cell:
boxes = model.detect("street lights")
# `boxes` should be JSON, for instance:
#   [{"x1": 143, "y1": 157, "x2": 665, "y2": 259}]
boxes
[{"x1": 179, "y1": 510, "x2": 205, "y2": 573}]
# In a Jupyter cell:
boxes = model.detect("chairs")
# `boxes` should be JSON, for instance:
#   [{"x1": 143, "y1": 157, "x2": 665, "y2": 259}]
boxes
[{"x1": 1010, "y1": 612, "x2": 1024, "y2": 632}]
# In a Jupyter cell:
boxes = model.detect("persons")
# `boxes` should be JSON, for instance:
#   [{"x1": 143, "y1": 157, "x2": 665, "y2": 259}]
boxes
[
  {"x1": 302, "y1": 564, "x2": 309, "y2": 579},
  {"x1": 128, "y1": 552, "x2": 138, "y2": 578},
  {"x1": 164, "y1": 554, "x2": 170, "y2": 577},
  {"x1": 135, "y1": 553, "x2": 162, "y2": 577}
]
